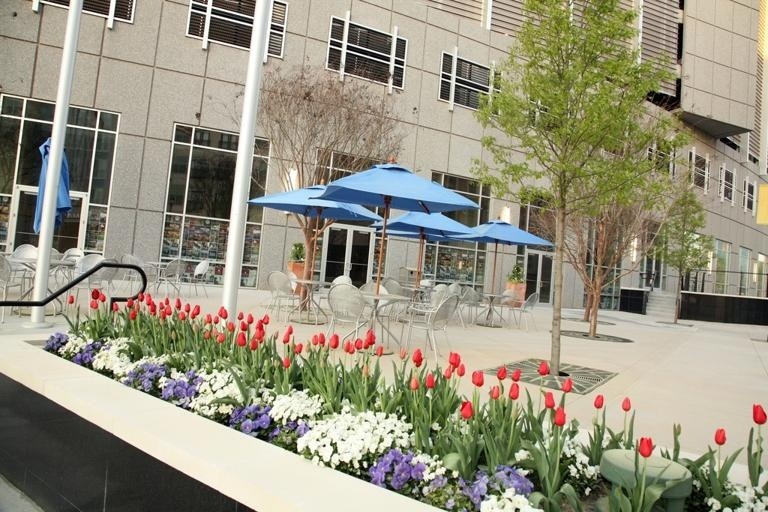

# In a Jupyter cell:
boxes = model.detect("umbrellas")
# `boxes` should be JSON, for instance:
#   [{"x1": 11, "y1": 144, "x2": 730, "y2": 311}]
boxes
[
  {"x1": 33, "y1": 137, "x2": 72, "y2": 235},
  {"x1": 246, "y1": 179, "x2": 384, "y2": 321},
  {"x1": 374, "y1": 229, "x2": 453, "y2": 244},
  {"x1": 369, "y1": 211, "x2": 476, "y2": 314},
  {"x1": 308, "y1": 157, "x2": 481, "y2": 348},
  {"x1": 445, "y1": 216, "x2": 557, "y2": 324}
]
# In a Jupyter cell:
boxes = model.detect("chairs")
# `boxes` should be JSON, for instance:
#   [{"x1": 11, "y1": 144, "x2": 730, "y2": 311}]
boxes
[
  {"x1": 461, "y1": 286, "x2": 540, "y2": 332},
  {"x1": 115, "y1": 254, "x2": 210, "y2": 299},
  {"x1": 324, "y1": 279, "x2": 466, "y2": 364},
  {"x1": 263, "y1": 271, "x2": 353, "y2": 327},
  {"x1": 2, "y1": 245, "x2": 116, "y2": 316}
]
